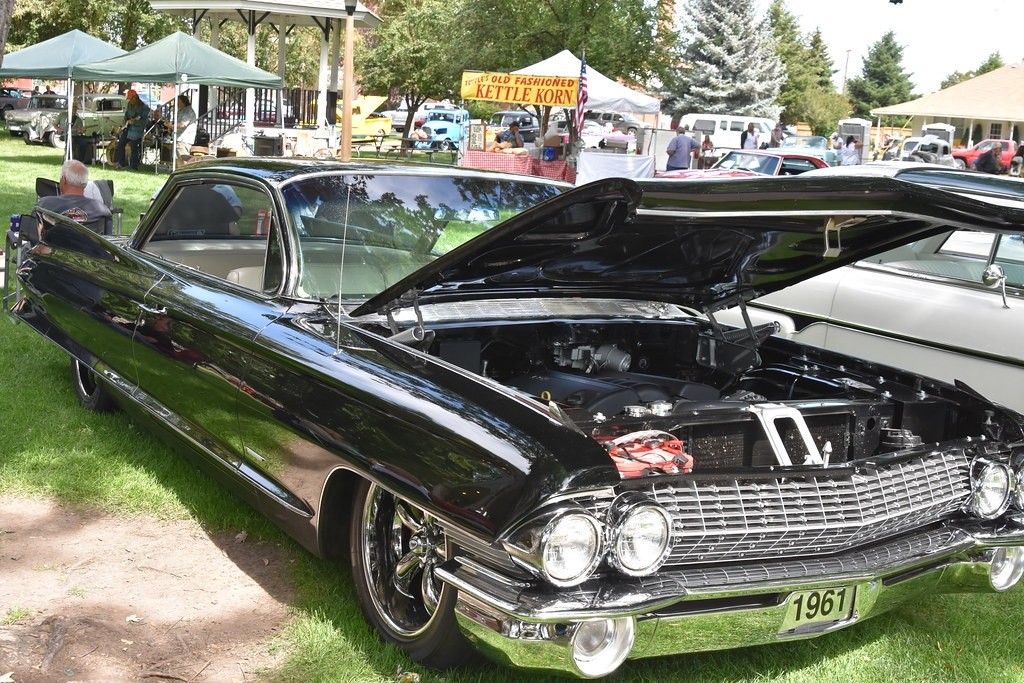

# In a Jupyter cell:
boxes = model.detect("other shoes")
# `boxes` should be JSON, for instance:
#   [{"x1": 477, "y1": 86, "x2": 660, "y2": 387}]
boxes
[
  {"x1": 131, "y1": 167, "x2": 139, "y2": 171},
  {"x1": 115, "y1": 164, "x2": 123, "y2": 169}
]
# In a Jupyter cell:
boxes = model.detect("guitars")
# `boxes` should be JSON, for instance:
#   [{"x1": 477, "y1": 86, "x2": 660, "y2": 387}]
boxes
[{"x1": 122, "y1": 116, "x2": 141, "y2": 130}]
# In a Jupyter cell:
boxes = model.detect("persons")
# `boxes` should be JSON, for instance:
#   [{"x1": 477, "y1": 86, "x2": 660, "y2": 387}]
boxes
[
  {"x1": 771, "y1": 122, "x2": 785, "y2": 148},
  {"x1": 841, "y1": 135, "x2": 863, "y2": 165},
  {"x1": 12, "y1": 160, "x2": 113, "y2": 271},
  {"x1": 281, "y1": 148, "x2": 334, "y2": 239},
  {"x1": 30, "y1": 85, "x2": 42, "y2": 108},
  {"x1": 830, "y1": 132, "x2": 843, "y2": 149},
  {"x1": 147, "y1": 165, "x2": 244, "y2": 238},
  {"x1": 666, "y1": 126, "x2": 700, "y2": 171},
  {"x1": 702, "y1": 135, "x2": 714, "y2": 151},
  {"x1": 881, "y1": 131, "x2": 910, "y2": 161},
  {"x1": 973, "y1": 140, "x2": 1005, "y2": 175},
  {"x1": 741, "y1": 122, "x2": 758, "y2": 149},
  {"x1": 42, "y1": 85, "x2": 58, "y2": 107},
  {"x1": 164, "y1": 95, "x2": 199, "y2": 174},
  {"x1": 871, "y1": 144, "x2": 879, "y2": 161},
  {"x1": 1008, "y1": 140, "x2": 1024, "y2": 177},
  {"x1": 496, "y1": 121, "x2": 524, "y2": 149},
  {"x1": 111, "y1": 89, "x2": 151, "y2": 170},
  {"x1": 148, "y1": 109, "x2": 171, "y2": 136},
  {"x1": 52, "y1": 105, "x2": 85, "y2": 137}
]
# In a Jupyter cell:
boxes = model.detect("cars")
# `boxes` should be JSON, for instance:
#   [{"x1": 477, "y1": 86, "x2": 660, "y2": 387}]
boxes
[
  {"x1": 0, "y1": 173, "x2": 1024, "y2": 680},
  {"x1": 4, "y1": 93, "x2": 128, "y2": 149},
  {"x1": 414, "y1": 108, "x2": 469, "y2": 151},
  {"x1": 785, "y1": 136, "x2": 1024, "y2": 292},
  {"x1": 486, "y1": 110, "x2": 540, "y2": 143},
  {"x1": 0, "y1": 87, "x2": 43, "y2": 120},
  {"x1": 652, "y1": 149, "x2": 831, "y2": 179},
  {"x1": 547, "y1": 113, "x2": 566, "y2": 129},
  {"x1": 133, "y1": 91, "x2": 171, "y2": 116},
  {"x1": 294, "y1": 95, "x2": 462, "y2": 142},
  {"x1": 711, "y1": 163, "x2": 1024, "y2": 422},
  {"x1": 218, "y1": 96, "x2": 294, "y2": 122}
]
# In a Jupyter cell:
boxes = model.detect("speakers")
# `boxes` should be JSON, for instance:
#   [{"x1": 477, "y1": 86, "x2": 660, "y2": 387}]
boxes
[
  {"x1": 254, "y1": 136, "x2": 283, "y2": 156},
  {"x1": 71, "y1": 135, "x2": 93, "y2": 164}
]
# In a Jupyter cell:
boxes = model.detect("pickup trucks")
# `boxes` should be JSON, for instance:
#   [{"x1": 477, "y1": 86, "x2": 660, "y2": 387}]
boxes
[{"x1": 949, "y1": 138, "x2": 1017, "y2": 170}]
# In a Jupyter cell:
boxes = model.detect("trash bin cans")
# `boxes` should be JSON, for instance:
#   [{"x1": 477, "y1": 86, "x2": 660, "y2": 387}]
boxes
[
  {"x1": 69, "y1": 135, "x2": 94, "y2": 165},
  {"x1": 254, "y1": 136, "x2": 284, "y2": 156}
]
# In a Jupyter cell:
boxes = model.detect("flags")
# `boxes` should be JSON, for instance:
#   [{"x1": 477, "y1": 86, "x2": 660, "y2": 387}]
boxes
[{"x1": 572, "y1": 54, "x2": 588, "y2": 136}]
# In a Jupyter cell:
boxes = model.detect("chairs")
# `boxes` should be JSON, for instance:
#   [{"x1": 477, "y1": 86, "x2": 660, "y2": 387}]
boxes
[
  {"x1": 3, "y1": 178, "x2": 124, "y2": 325},
  {"x1": 228, "y1": 264, "x2": 387, "y2": 298},
  {"x1": 380, "y1": 263, "x2": 431, "y2": 286}
]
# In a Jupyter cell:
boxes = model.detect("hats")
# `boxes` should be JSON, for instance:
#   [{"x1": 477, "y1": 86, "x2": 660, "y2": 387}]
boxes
[{"x1": 125, "y1": 90, "x2": 136, "y2": 100}]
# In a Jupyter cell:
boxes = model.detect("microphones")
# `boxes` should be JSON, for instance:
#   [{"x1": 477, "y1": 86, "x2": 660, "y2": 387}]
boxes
[{"x1": 100, "y1": 98, "x2": 106, "y2": 105}]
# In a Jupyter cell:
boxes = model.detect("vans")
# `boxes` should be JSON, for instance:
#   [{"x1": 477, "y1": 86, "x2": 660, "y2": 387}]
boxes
[{"x1": 678, "y1": 112, "x2": 777, "y2": 149}]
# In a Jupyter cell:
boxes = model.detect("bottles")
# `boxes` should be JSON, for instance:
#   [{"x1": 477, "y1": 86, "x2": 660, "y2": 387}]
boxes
[
  {"x1": 636, "y1": 145, "x2": 642, "y2": 155},
  {"x1": 10, "y1": 212, "x2": 21, "y2": 232}
]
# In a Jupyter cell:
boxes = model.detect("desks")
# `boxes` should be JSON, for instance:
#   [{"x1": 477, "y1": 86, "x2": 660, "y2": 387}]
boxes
[
  {"x1": 462, "y1": 150, "x2": 577, "y2": 184},
  {"x1": 347, "y1": 134, "x2": 397, "y2": 158},
  {"x1": 396, "y1": 138, "x2": 459, "y2": 165}
]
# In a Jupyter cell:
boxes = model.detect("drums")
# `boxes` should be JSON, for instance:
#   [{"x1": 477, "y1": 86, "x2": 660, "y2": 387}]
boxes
[
  {"x1": 106, "y1": 141, "x2": 132, "y2": 166},
  {"x1": 109, "y1": 126, "x2": 122, "y2": 139}
]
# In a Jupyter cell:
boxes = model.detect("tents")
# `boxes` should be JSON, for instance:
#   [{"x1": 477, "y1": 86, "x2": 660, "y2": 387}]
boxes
[
  {"x1": 0, "y1": 26, "x2": 131, "y2": 162},
  {"x1": 72, "y1": 31, "x2": 287, "y2": 173},
  {"x1": 458, "y1": 49, "x2": 661, "y2": 185}
]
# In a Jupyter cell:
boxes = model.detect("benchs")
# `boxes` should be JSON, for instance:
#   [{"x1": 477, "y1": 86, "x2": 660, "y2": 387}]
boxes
[
  {"x1": 352, "y1": 143, "x2": 371, "y2": 146},
  {"x1": 383, "y1": 147, "x2": 458, "y2": 155},
  {"x1": 146, "y1": 239, "x2": 440, "y2": 280}
]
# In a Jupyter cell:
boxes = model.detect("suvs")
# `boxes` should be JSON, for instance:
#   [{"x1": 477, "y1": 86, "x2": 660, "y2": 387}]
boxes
[{"x1": 583, "y1": 110, "x2": 650, "y2": 136}]
